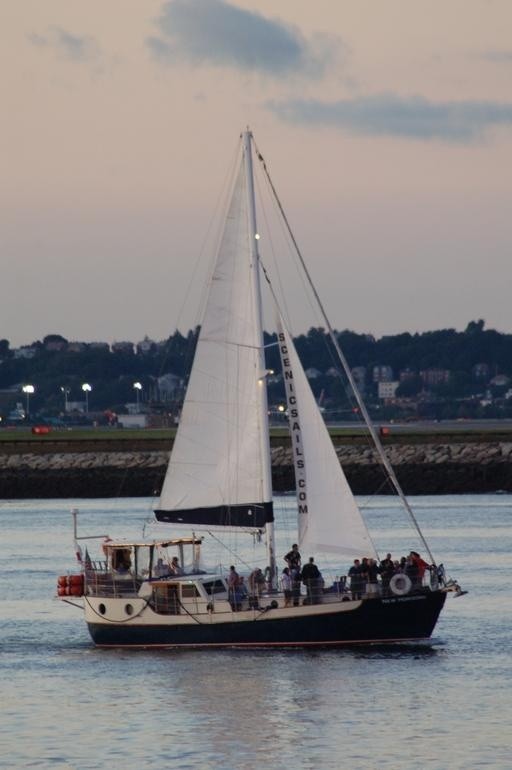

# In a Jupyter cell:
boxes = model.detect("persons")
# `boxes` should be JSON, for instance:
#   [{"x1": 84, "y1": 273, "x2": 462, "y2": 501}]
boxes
[
  {"x1": 117, "y1": 550, "x2": 132, "y2": 573},
  {"x1": 227, "y1": 564, "x2": 266, "y2": 611},
  {"x1": 278, "y1": 544, "x2": 325, "y2": 608},
  {"x1": 152, "y1": 558, "x2": 172, "y2": 577},
  {"x1": 170, "y1": 557, "x2": 182, "y2": 574},
  {"x1": 347, "y1": 550, "x2": 432, "y2": 600}
]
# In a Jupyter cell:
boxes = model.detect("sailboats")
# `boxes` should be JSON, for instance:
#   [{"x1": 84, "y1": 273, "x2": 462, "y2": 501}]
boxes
[{"x1": 56, "y1": 125, "x2": 468, "y2": 648}]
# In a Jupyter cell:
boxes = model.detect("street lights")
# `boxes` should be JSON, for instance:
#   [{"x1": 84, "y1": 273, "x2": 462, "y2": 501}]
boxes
[
  {"x1": 22, "y1": 385, "x2": 34, "y2": 422},
  {"x1": 60, "y1": 387, "x2": 71, "y2": 412},
  {"x1": 133, "y1": 381, "x2": 143, "y2": 415},
  {"x1": 82, "y1": 383, "x2": 92, "y2": 413}
]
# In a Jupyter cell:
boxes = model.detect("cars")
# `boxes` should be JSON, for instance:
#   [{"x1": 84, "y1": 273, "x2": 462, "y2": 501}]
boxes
[
  {"x1": 270, "y1": 402, "x2": 327, "y2": 423},
  {"x1": 0, "y1": 410, "x2": 72, "y2": 434}
]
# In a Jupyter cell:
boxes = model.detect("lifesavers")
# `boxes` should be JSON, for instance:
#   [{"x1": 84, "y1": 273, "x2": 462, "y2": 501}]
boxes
[
  {"x1": 58, "y1": 576, "x2": 82, "y2": 596},
  {"x1": 390, "y1": 573, "x2": 412, "y2": 595}
]
